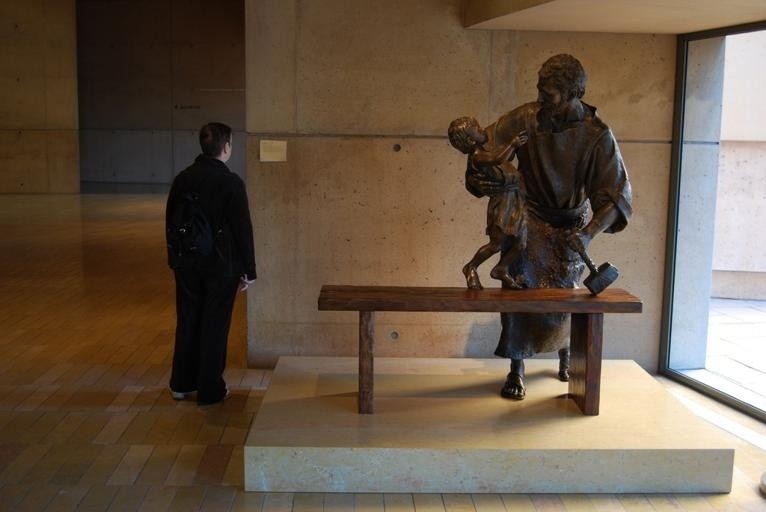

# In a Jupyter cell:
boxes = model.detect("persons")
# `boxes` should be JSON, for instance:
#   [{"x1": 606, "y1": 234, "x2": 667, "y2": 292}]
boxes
[
  {"x1": 463, "y1": 52, "x2": 634, "y2": 400},
  {"x1": 446, "y1": 116, "x2": 529, "y2": 291},
  {"x1": 163, "y1": 120, "x2": 258, "y2": 405}
]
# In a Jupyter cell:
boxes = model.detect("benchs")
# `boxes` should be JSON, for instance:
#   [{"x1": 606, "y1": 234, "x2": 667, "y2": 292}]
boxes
[{"x1": 318, "y1": 284, "x2": 644, "y2": 416}]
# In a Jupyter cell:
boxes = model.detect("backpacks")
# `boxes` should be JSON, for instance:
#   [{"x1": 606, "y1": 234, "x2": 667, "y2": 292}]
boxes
[{"x1": 166, "y1": 165, "x2": 229, "y2": 292}]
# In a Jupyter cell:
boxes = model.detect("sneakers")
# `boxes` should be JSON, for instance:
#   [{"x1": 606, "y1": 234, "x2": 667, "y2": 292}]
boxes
[
  {"x1": 166, "y1": 384, "x2": 188, "y2": 400},
  {"x1": 198, "y1": 390, "x2": 233, "y2": 406}
]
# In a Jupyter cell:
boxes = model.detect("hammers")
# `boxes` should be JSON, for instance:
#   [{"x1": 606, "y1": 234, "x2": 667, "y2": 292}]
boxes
[{"x1": 565, "y1": 229, "x2": 618, "y2": 296}]
